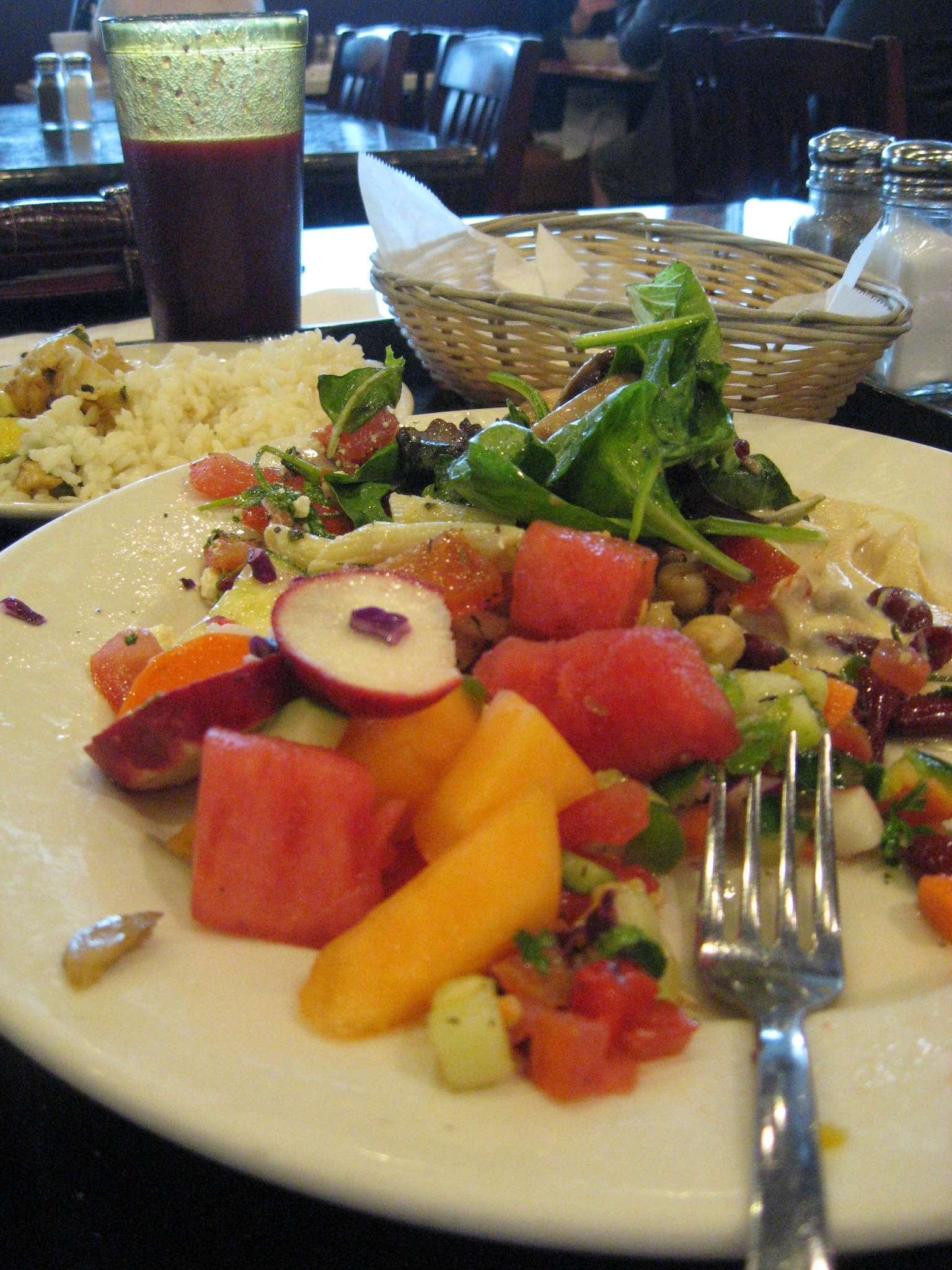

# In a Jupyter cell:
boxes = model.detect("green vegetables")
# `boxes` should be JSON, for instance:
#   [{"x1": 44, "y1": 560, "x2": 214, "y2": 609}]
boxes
[{"x1": 196, "y1": 260, "x2": 951, "y2": 989}]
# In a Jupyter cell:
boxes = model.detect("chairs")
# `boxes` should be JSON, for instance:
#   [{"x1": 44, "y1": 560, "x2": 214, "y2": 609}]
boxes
[
  {"x1": 0, "y1": 184, "x2": 151, "y2": 336},
  {"x1": 329, "y1": 28, "x2": 543, "y2": 218},
  {"x1": 666, "y1": 27, "x2": 908, "y2": 201}
]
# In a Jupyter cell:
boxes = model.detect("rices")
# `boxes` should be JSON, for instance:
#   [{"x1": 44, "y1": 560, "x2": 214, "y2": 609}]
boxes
[{"x1": 1, "y1": 324, "x2": 380, "y2": 505}]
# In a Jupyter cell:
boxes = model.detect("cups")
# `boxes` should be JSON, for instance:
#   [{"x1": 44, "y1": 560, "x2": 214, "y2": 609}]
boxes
[{"x1": 98, "y1": 10, "x2": 309, "y2": 339}]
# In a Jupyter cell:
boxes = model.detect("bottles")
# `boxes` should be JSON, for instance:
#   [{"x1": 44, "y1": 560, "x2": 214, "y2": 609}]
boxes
[
  {"x1": 853, "y1": 140, "x2": 952, "y2": 400},
  {"x1": 62, "y1": 52, "x2": 96, "y2": 131},
  {"x1": 784, "y1": 125, "x2": 897, "y2": 264},
  {"x1": 31, "y1": 52, "x2": 67, "y2": 131}
]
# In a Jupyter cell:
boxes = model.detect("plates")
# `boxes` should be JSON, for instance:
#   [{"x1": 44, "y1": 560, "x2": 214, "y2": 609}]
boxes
[
  {"x1": 0, "y1": 339, "x2": 414, "y2": 540},
  {"x1": 0, "y1": 414, "x2": 950, "y2": 1257}
]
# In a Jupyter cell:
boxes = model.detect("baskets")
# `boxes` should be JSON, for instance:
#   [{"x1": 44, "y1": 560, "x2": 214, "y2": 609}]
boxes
[{"x1": 368, "y1": 210, "x2": 914, "y2": 422}]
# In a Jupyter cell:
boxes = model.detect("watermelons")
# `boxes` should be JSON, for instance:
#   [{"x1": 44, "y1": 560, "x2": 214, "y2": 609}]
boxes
[{"x1": 90, "y1": 405, "x2": 737, "y2": 1096}]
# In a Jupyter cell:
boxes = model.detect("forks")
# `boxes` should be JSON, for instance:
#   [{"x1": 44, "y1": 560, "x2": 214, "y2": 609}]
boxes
[{"x1": 692, "y1": 724, "x2": 847, "y2": 1270}]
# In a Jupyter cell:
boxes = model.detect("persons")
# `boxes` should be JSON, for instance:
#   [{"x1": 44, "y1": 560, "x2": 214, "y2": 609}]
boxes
[{"x1": 521, "y1": 0, "x2": 817, "y2": 208}]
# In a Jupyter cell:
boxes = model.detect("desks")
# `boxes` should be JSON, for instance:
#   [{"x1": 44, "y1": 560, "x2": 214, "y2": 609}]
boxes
[{"x1": 0, "y1": 93, "x2": 486, "y2": 267}]
